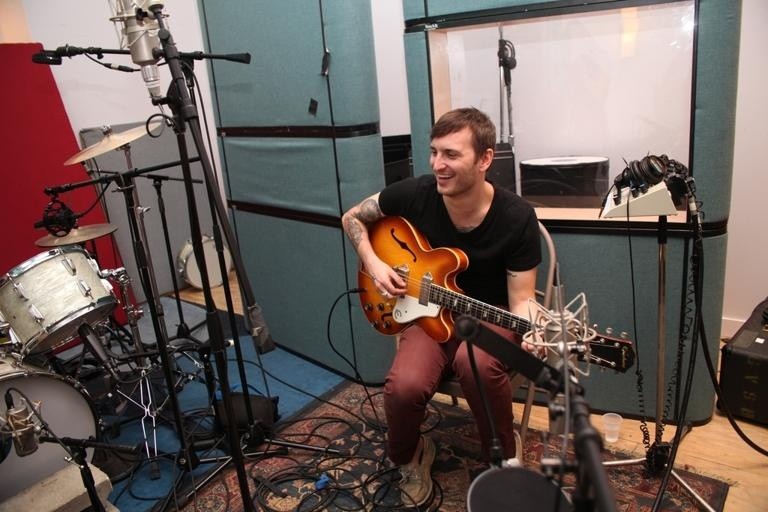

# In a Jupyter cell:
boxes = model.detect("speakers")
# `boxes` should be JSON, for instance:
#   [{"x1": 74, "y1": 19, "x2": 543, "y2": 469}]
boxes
[{"x1": 485, "y1": 143, "x2": 516, "y2": 193}]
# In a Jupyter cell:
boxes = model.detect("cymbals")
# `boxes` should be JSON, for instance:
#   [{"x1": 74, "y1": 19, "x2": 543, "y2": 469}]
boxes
[
  {"x1": 34, "y1": 223, "x2": 118, "y2": 247},
  {"x1": 63, "y1": 121, "x2": 160, "y2": 167}
]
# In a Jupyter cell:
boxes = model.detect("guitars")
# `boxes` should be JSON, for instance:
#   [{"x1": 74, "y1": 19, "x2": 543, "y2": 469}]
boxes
[{"x1": 358, "y1": 217, "x2": 637, "y2": 373}]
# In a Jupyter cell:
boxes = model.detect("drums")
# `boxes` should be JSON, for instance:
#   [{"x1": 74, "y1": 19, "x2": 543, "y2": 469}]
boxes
[
  {"x1": 176, "y1": 233, "x2": 230, "y2": 288},
  {"x1": 0, "y1": 350, "x2": 97, "y2": 502},
  {"x1": 0, "y1": 245, "x2": 114, "y2": 355}
]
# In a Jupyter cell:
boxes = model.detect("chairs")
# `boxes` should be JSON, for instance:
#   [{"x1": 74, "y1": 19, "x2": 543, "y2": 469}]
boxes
[{"x1": 395, "y1": 217, "x2": 555, "y2": 452}]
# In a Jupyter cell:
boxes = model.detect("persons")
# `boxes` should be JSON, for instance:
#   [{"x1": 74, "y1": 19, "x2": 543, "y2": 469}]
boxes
[{"x1": 339, "y1": 106, "x2": 591, "y2": 506}]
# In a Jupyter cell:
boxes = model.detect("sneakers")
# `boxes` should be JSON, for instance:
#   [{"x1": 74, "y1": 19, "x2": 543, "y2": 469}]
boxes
[
  {"x1": 491, "y1": 429, "x2": 523, "y2": 468},
  {"x1": 401, "y1": 435, "x2": 435, "y2": 508}
]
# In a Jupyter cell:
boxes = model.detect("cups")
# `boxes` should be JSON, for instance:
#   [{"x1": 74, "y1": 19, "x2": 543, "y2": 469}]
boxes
[{"x1": 602, "y1": 412, "x2": 623, "y2": 443}]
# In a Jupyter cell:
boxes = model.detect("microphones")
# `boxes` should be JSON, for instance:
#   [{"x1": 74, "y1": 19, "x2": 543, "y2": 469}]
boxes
[
  {"x1": 9, "y1": 409, "x2": 39, "y2": 458},
  {"x1": 34, "y1": 198, "x2": 74, "y2": 237},
  {"x1": 31, "y1": 53, "x2": 62, "y2": 65},
  {"x1": 109, "y1": 0, "x2": 162, "y2": 99}
]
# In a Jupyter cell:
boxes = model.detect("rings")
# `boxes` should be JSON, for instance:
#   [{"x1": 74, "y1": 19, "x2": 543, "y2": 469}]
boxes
[{"x1": 381, "y1": 290, "x2": 387, "y2": 296}]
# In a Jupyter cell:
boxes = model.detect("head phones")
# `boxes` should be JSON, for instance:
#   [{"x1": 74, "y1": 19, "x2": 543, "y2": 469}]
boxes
[
  {"x1": 614, "y1": 154, "x2": 668, "y2": 187},
  {"x1": 497, "y1": 39, "x2": 516, "y2": 68}
]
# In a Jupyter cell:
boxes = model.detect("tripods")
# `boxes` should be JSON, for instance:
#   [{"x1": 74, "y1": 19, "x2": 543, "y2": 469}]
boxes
[{"x1": 542, "y1": 217, "x2": 720, "y2": 512}]
